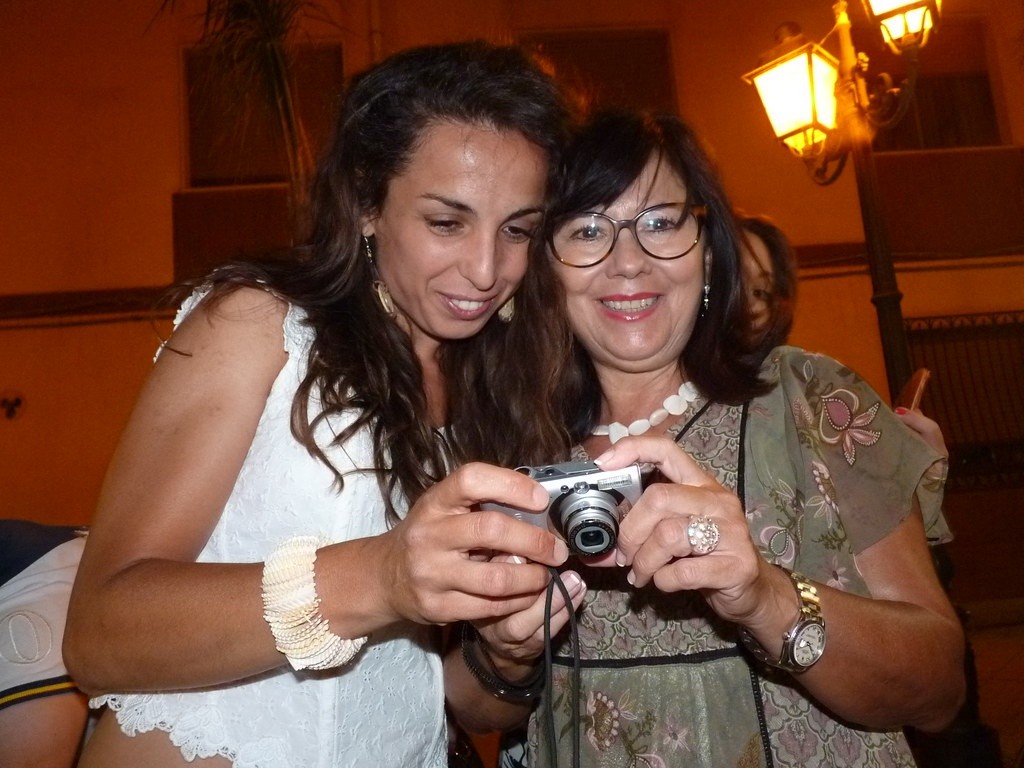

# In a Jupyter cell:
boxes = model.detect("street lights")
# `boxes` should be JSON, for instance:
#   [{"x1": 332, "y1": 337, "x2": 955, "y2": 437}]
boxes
[{"x1": 740, "y1": 1, "x2": 1006, "y2": 768}]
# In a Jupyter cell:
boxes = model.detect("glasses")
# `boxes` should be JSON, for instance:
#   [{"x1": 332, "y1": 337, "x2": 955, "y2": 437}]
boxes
[{"x1": 545, "y1": 202, "x2": 709, "y2": 267}]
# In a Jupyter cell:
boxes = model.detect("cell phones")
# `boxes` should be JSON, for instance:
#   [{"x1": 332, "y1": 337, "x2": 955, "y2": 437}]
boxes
[{"x1": 893, "y1": 368, "x2": 930, "y2": 412}]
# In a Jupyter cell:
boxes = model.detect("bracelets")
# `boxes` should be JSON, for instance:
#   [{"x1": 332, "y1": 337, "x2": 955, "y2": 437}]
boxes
[{"x1": 261, "y1": 534, "x2": 368, "y2": 673}]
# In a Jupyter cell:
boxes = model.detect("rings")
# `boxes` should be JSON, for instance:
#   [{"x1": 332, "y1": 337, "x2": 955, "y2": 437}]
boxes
[{"x1": 688, "y1": 514, "x2": 720, "y2": 557}]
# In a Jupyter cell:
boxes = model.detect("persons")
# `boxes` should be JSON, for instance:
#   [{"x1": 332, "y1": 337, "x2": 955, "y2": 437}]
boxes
[
  {"x1": 732, "y1": 210, "x2": 949, "y2": 461},
  {"x1": 64, "y1": 37, "x2": 586, "y2": 768},
  {"x1": 445, "y1": 109, "x2": 968, "y2": 767}
]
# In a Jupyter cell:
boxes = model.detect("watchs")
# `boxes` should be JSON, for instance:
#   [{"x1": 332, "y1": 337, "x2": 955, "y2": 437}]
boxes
[{"x1": 736, "y1": 562, "x2": 827, "y2": 673}]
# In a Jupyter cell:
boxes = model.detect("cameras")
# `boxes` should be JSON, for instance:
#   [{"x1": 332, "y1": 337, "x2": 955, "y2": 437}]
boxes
[{"x1": 480, "y1": 460, "x2": 644, "y2": 559}]
